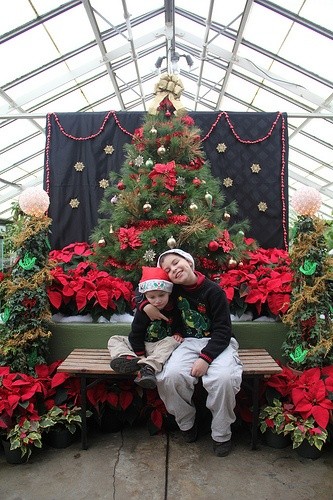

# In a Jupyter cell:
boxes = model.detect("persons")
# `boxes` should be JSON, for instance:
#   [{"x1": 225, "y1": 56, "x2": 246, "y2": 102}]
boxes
[
  {"x1": 107, "y1": 265, "x2": 185, "y2": 390},
  {"x1": 134, "y1": 248, "x2": 244, "y2": 458}
]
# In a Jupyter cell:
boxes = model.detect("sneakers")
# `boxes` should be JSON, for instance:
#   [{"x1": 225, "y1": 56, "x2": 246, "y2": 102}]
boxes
[
  {"x1": 134, "y1": 364, "x2": 157, "y2": 389},
  {"x1": 110, "y1": 355, "x2": 144, "y2": 373}
]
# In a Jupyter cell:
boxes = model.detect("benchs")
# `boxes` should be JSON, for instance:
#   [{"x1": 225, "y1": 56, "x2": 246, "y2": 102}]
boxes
[{"x1": 56, "y1": 348, "x2": 283, "y2": 451}]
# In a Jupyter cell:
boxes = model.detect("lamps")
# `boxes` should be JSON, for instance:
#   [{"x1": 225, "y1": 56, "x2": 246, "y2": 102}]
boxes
[
  {"x1": 155, "y1": 56, "x2": 167, "y2": 68},
  {"x1": 179, "y1": 54, "x2": 194, "y2": 67}
]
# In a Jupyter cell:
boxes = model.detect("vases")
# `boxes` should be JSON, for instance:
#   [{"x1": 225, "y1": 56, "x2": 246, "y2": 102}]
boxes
[
  {"x1": 101, "y1": 414, "x2": 120, "y2": 433},
  {"x1": 262, "y1": 428, "x2": 291, "y2": 449},
  {"x1": 48, "y1": 430, "x2": 68, "y2": 449},
  {"x1": 1, "y1": 436, "x2": 22, "y2": 464},
  {"x1": 295, "y1": 438, "x2": 324, "y2": 460}
]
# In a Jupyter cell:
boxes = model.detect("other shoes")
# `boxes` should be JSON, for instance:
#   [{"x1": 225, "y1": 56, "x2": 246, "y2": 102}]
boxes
[
  {"x1": 181, "y1": 423, "x2": 197, "y2": 442},
  {"x1": 215, "y1": 440, "x2": 232, "y2": 457}
]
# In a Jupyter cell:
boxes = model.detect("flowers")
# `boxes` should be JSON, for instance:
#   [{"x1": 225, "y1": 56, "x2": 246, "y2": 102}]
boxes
[
  {"x1": 45, "y1": 241, "x2": 295, "y2": 323},
  {"x1": 0, "y1": 358, "x2": 333, "y2": 463}
]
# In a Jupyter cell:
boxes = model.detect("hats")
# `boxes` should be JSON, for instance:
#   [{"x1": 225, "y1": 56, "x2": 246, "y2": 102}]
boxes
[
  {"x1": 138, "y1": 266, "x2": 173, "y2": 293},
  {"x1": 157, "y1": 249, "x2": 194, "y2": 271}
]
